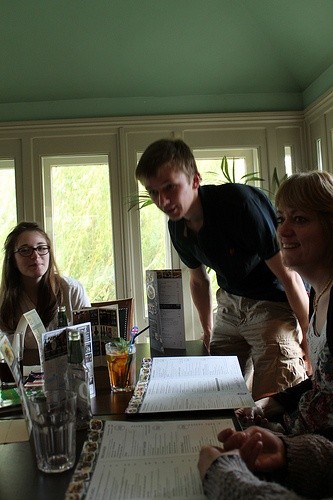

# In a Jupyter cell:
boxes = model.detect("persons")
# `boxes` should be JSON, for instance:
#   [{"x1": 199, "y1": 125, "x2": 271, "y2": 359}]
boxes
[
  {"x1": 198, "y1": 171, "x2": 333, "y2": 500},
  {"x1": 135, "y1": 138, "x2": 312, "y2": 401},
  {"x1": 0, "y1": 221, "x2": 90, "y2": 350}
]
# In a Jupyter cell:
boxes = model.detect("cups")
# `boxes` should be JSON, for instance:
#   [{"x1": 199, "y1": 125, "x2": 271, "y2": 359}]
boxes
[
  {"x1": 105, "y1": 342, "x2": 136, "y2": 393},
  {"x1": 21, "y1": 388, "x2": 77, "y2": 473}
]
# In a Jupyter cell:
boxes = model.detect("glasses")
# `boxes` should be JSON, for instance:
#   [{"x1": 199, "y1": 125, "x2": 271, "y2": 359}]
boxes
[{"x1": 13, "y1": 245, "x2": 49, "y2": 257}]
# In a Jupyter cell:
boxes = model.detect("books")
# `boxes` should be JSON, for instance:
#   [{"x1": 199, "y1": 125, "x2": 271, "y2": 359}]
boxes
[
  {"x1": 145, "y1": 269, "x2": 187, "y2": 355},
  {"x1": 65, "y1": 413, "x2": 266, "y2": 500},
  {"x1": 125, "y1": 355, "x2": 257, "y2": 414}
]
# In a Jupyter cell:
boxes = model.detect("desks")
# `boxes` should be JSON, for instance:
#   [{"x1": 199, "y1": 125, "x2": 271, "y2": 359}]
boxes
[{"x1": 0, "y1": 339, "x2": 273, "y2": 500}]
